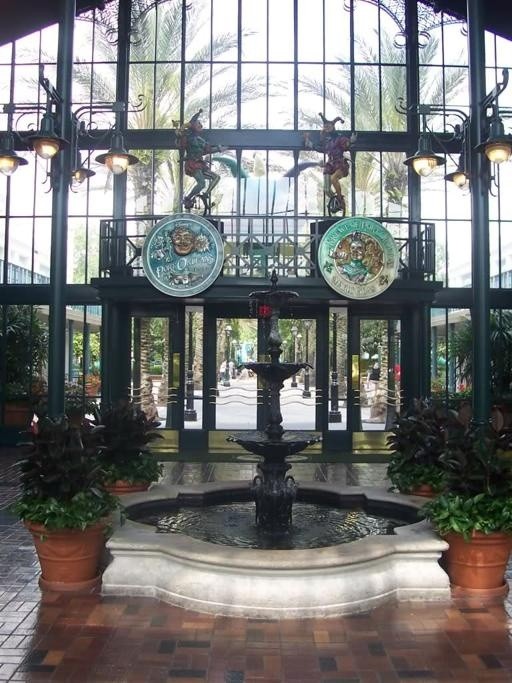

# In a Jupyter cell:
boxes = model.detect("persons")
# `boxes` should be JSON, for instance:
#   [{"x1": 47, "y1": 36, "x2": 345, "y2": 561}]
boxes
[
  {"x1": 367, "y1": 362, "x2": 380, "y2": 396},
  {"x1": 176, "y1": 109, "x2": 220, "y2": 209},
  {"x1": 338, "y1": 232, "x2": 379, "y2": 284},
  {"x1": 304, "y1": 113, "x2": 357, "y2": 211},
  {"x1": 169, "y1": 227, "x2": 195, "y2": 256}
]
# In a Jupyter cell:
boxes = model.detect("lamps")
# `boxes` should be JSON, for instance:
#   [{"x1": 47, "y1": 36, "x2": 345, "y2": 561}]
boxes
[
  {"x1": 0, "y1": 100, "x2": 138, "y2": 183},
  {"x1": 404, "y1": 105, "x2": 511, "y2": 189}
]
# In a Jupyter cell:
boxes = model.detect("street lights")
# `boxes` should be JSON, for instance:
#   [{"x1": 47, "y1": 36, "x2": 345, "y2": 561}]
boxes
[
  {"x1": 301, "y1": 319, "x2": 313, "y2": 398},
  {"x1": 394, "y1": 67, "x2": 512, "y2": 427},
  {"x1": 0, "y1": 64, "x2": 149, "y2": 424},
  {"x1": 224, "y1": 325, "x2": 232, "y2": 387},
  {"x1": 291, "y1": 326, "x2": 299, "y2": 387}
]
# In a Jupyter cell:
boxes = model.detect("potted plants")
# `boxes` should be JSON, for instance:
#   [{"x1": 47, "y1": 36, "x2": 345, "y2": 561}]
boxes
[
  {"x1": 2, "y1": 306, "x2": 46, "y2": 431},
  {"x1": 19, "y1": 412, "x2": 122, "y2": 590},
  {"x1": 98, "y1": 399, "x2": 166, "y2": 495},
  {"x1": 384, "y1": 395, "x2": 511, "y2": 598}
]
